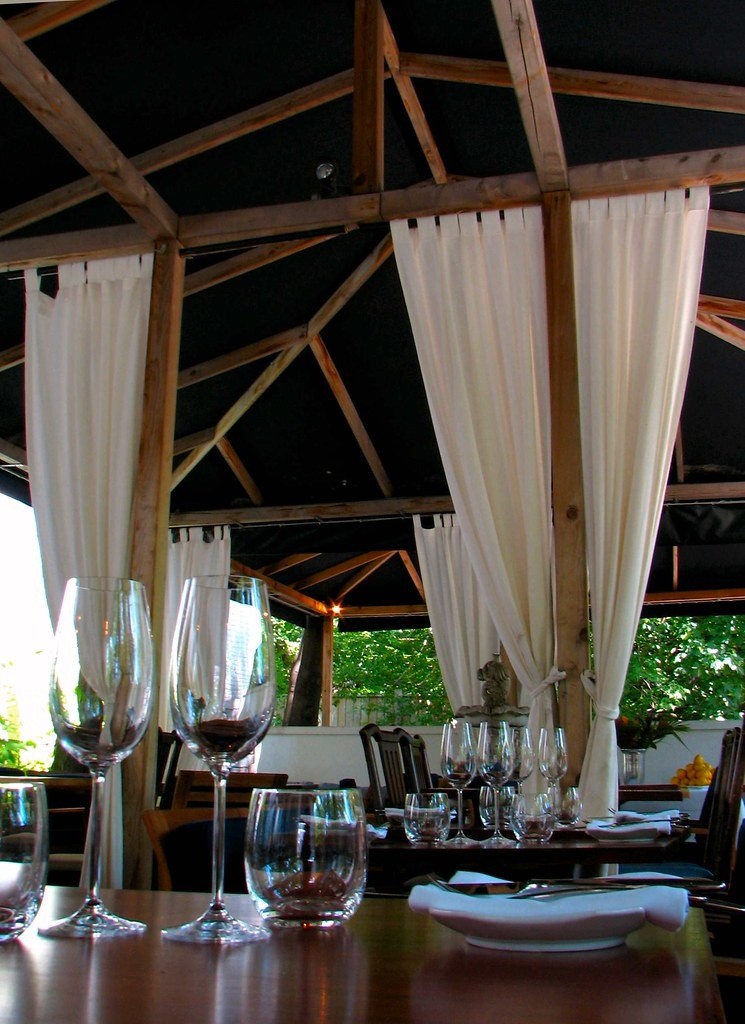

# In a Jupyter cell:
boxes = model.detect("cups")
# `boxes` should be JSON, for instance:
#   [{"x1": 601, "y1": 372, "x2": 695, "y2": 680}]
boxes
[
  {"x1": 509, "y1": 793, "x2": 555, "y2": 841},
  {"x1": 547, "y1": 786, "x2": 583, "y2": 828},
  {"x1": 244, "y1": 787, "x2": 370, "y2": 927},
  {"x1": 403, "y1": 793, "x2": 452, "y2": 846},
  {"x1": 0, "y1": 782, "x2": 51, "y2": 943},
  {"x1": 479, "y1": 786, "x2": 515, "y2": 827}
]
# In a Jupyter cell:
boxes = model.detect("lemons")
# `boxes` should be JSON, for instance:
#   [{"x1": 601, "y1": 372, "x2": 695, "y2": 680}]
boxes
[{"x1": 671, "y1": 754, "x2": 714, "y2": 787}]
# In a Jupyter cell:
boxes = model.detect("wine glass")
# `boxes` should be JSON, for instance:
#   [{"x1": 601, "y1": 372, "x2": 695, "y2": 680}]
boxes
[
  {"x1": 38, "y1": 575, "x2": 154, "y2": 939},
  {"x1": 440, "y1": 720, "x2": 568, "y2": 846},
  {"x1": 160, "y1": 573, "x2": 278, "y2": 944}
]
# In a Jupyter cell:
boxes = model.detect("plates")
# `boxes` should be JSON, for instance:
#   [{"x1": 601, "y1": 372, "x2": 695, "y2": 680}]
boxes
[
  {"x1": 428, "y1": 908, "x2": 647, "y2": 953},
  {"x1": 586, "y1": 826, "x2": 660, "y2": 843},
  {"x1": 680, "y1": 785, "x2": 710, "y2": 788}
]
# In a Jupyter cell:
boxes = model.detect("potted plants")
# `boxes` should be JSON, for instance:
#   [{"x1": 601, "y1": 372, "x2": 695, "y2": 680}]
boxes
[{"x1": 614, "y1": 701, "x2": 692, "y2": 784}]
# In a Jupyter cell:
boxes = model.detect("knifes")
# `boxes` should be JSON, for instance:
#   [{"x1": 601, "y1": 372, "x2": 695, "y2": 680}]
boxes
[{"x1": 444, "y1": 878, "x2": 725, "y2": 894}]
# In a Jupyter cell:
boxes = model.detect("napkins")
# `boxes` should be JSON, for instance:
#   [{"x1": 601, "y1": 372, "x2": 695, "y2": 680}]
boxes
[
  {"x1": 586, "y1": 807, "x2": 683, "y2": 837},
  {"x1": 409, "y1": 868, "x2": 691, "y2": 934}
]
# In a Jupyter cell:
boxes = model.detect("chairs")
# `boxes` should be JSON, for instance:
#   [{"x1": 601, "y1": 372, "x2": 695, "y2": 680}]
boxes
[{"x1": 139, "y1": 722, "x2": 439, "y2": 898}]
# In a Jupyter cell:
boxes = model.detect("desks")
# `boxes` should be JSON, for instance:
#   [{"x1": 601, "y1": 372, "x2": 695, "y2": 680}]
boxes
[
  {"x1": 368, "y1": 827, "x2": 691, "y2": 888},
  {"x1": 0, "y1": 886, "x2": 728, "y2": 1024}
]
